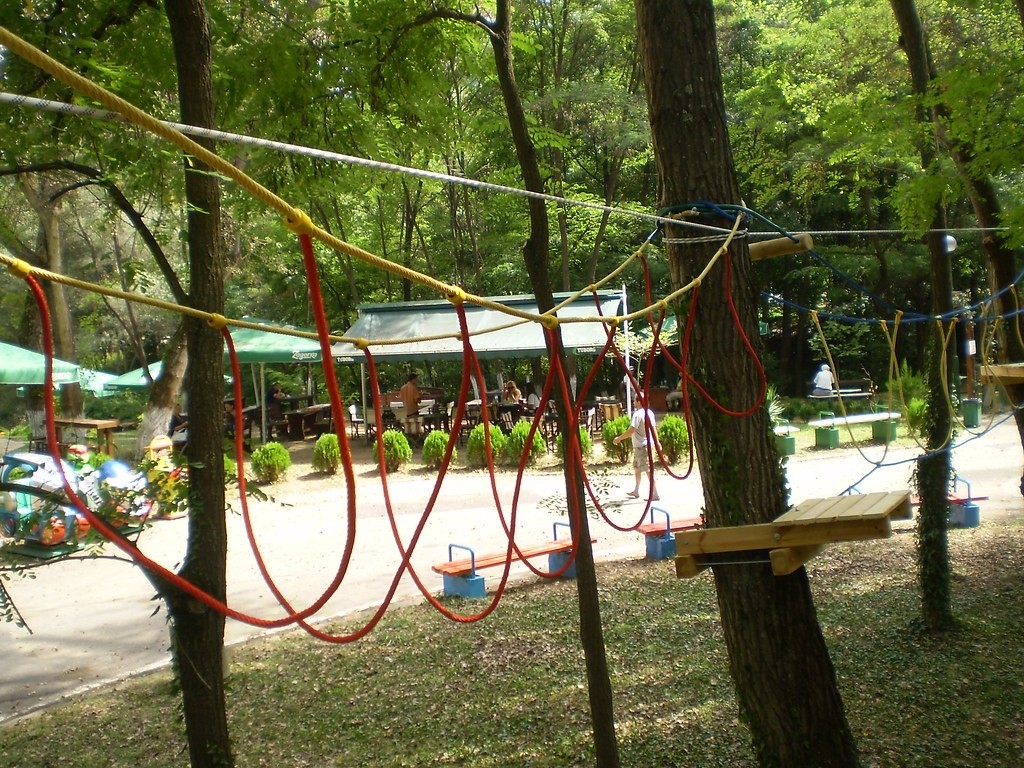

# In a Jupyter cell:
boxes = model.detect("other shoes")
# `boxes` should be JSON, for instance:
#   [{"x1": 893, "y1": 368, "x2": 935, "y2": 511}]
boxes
[
  {"x1": 627, "y1": 491, "x2": 640, "y2": 498},
  {"x1": 644, "y1": 495, "x2": 659, "y2": 501}
]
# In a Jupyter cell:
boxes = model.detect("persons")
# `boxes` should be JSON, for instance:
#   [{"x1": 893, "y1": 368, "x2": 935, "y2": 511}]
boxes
[
  {"x1": 613, "y1": 391, "x2": 662, "y2": 501},
  {"x1": 813, "y1": 364, "x2": 835, "y2": 396},
  {"x1": 620, "y1": 366, "x2": 683, "y2": 411},
  {"x1": 512, "y1": 382, "x2": 541, "y2": 425},
  {"x1": 505, "y1": 381, "x2": 521, "y2": 404},
  {"x1": 167, "y1": 382, "x2": 292, "y2": 442},
  {"x1": 399, "y1": 374, "x2": 421, "y2": 438}
]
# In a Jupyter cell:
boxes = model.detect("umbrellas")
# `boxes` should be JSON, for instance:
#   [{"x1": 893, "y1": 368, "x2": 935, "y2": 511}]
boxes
[
  {"x1": 636, "y1": 315, "x2": 770, "y2": 346},
  {"x1": 104, "y1": 360, "x2": 234, "y2": 393},
  {"x1": 0, "y1": 341, "x2": 120, "y2": 397},
  {"x1": 224, "y1": 315, "x2": 334, "y2": 445}
]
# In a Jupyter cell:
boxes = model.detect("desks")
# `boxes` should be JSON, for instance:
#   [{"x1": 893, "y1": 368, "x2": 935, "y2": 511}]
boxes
[
  {"x1": 40, "y1": 417, "x2": 120, "y2": 457},
  {"x1": 640, "y1": 385, "x2": 668, "y2": 413},
  {"x1": 280, "y1": 402, "x2": 332, "y2": 440},
  {"x1": 806, "y1": 377, "x2": 874, "y2": 398}
]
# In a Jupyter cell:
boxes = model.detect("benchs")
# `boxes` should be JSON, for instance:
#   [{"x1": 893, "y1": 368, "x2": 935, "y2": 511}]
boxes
[
  {"x1": 630, "y1": 506, "x2": 706, "y2": 560},
  {"x1": 431, "y1": 523, "x2": 597, "y2": 599},
  {"x1": 805, "y1": 410, "x2": 902, "y2": 450},
  {"x1": 908, "y1": 477, "x2": 981, "y2": 528},
  {"x1": 313, "y1": 418, "x2": 335, "y2": 441},
  {"x1": 28, "y1": 436, "x2": 97, "y2": 460},
  {"x1": 772, "y1": 419, "x2": 801, "y2": 459},
  {"x1": 273, "y1": 418, "x2": 288, "y2": 437},
  {"x1": 807, "y1": 387, "x2": 875, "y2": 412}
]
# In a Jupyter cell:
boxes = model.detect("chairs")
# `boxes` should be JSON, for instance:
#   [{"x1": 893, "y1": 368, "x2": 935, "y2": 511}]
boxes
[{"x1": 343, "y1": 396, "x2": 628, "y2": 456}]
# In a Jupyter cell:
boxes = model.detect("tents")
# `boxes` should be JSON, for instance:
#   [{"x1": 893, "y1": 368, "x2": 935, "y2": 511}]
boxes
[{"x1": 330, "y1": 284, "x2": 631, "y2": 445}]
